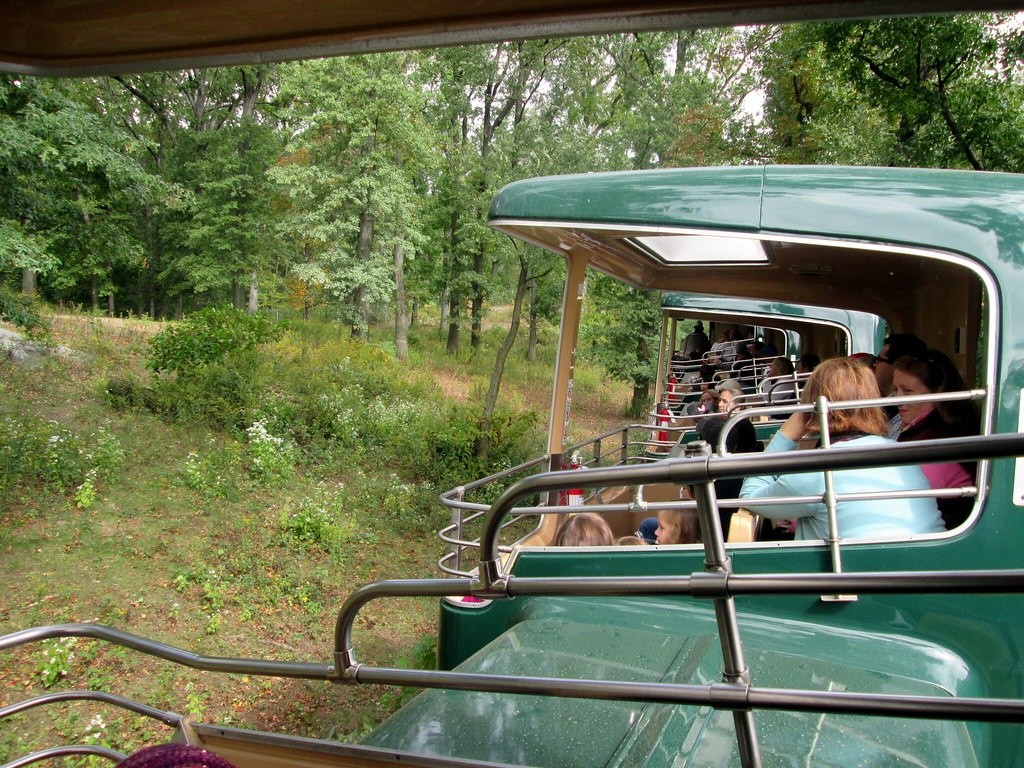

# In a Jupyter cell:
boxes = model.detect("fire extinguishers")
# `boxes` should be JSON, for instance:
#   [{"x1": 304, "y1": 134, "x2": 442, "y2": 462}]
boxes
[
  {"x1": 566, "y1": 451, "x2": 584, "y2": 517},
  {"x1": 667, "y1": 373, "x2": 677, "y2": 399},
  {"x1": 659, "y1": 401, "x2": 670, "y2": 441}
]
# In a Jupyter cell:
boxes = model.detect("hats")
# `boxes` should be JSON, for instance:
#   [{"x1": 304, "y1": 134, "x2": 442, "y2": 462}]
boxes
[
  {"x1": 693, "y1": 320, "x2": 704, "y2": 330},
  {"x1": 715, "y1": 379, "x2": 741, "y2": 390}
]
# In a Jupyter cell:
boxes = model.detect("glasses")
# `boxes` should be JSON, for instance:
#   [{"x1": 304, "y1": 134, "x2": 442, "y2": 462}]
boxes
[{"x1": 872, "y1": 356, "x2": 895, "y2": 368}]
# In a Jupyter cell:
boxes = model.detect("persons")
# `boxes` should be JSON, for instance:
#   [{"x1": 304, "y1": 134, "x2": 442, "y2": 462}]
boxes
[
  {"x1": 851, "y1": 334, "x2": 982, "y2": 530},
  {"x1": 677, "y1": 321, "x2": 755, "y2": 366},
  {"x1": 555, "y1": 367, "x2": 759, "y2": 545},
  {"x1": 739, "y1": 337, "x2": 946, "y2": 544}
]
